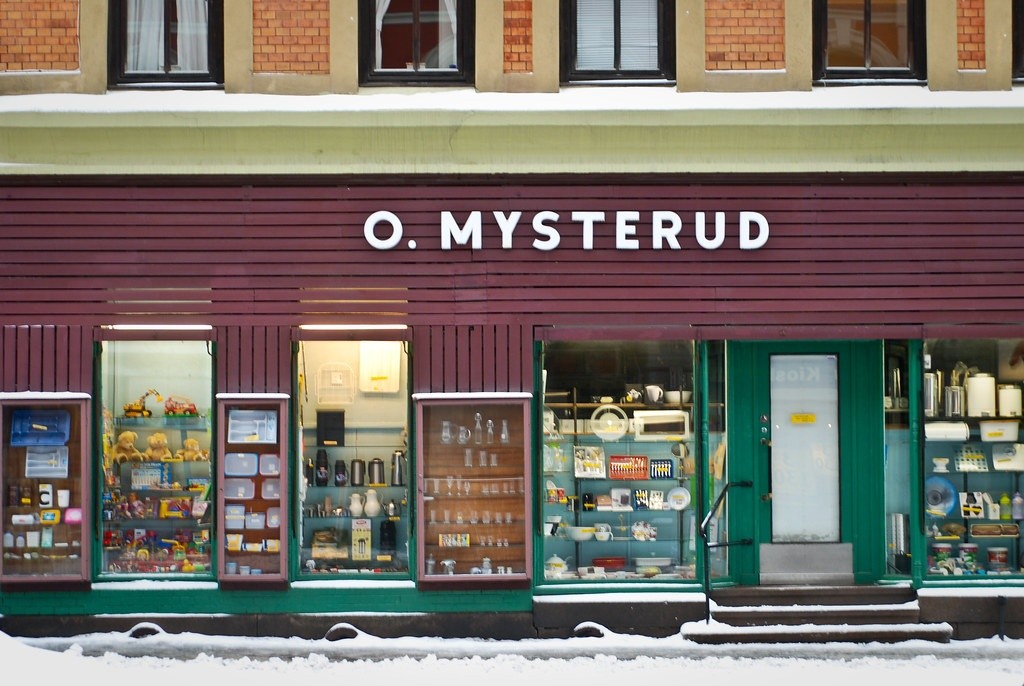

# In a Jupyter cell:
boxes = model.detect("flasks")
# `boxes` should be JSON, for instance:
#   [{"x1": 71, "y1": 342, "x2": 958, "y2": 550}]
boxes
[
  {"x1": 350, "y1": 459, "x2": 366, "y2": 487},
  {"x1": 368, "y1": 458, "x2": 385, "y2": 484},
  {"x1": 316, "y1": 449, "x2": 328, "y2": 487},
  {"x1": 391, "y1": 450, "x2": 408, "y2": 486}
]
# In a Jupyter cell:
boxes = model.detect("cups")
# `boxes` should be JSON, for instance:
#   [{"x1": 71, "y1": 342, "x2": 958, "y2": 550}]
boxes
[
  {"x1": 57, "y1": 490, "x2": 70, "y2": 508},
  {"x1": 428, "y1": 508, "x2": 512, "y2": 526},
  {"x1": 646, "y1": 385, "x2": 663, "y2": 402},
  {"x1": 593, "y1": 523, "x2": 614, "y2": 542},
  {"x1": 226, "y1": 562, "x2": 236, "y2": 574},
  {"x1": 334, "y1": 460, "x2": 346, "y2": 486},
  {"x1": 239, "y1": 566, "x2": 250, "y2": 576},
  {"x1": 479, "y1": 535, "x2": 510, "y2": 547},
  {"x1": 26, "y1": 531, "x2": 41, "y2": 548},
  {"x1": 578, "y1": 567, "x2": 588, "y2": 578},
  {"x1": 251, "y1": 569, "x2": 263, "y2": 576},
  {"x1": 479, "y1": 477, "x2": 524, "y2": 497},
  {"x1": 462, "y1": 447, "x2": 499, "y2": 467}
]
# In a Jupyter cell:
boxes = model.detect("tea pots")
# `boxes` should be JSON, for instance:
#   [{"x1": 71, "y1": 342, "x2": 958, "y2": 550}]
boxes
[{"x1": 381, "y1": 499, "x2": 402, "y2": 518}]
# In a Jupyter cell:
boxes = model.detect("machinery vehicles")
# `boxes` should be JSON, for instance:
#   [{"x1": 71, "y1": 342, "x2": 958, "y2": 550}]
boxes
[
  {"x1": 162, "y1": 393, "x2": 198, "y2": 416},
  {"x1": 122, "y1": 388, "x2": 164, "y2": 419}
]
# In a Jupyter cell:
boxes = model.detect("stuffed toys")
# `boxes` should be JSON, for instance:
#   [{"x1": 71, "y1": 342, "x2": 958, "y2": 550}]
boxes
[
  {"x1": 113, "y1": 431, "x2": 143, "y2": 464},
  {"x1": 142, "y1": 433, "x2": 172, "y2": 461},
  {"x1": 177, "y1": 439, "x2": 208, "y2": 460}
]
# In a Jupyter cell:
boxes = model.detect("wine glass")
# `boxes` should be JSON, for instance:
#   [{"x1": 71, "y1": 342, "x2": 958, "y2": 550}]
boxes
[{"x1": 422, "y1": 473, "x2": 472, "y2": 497}]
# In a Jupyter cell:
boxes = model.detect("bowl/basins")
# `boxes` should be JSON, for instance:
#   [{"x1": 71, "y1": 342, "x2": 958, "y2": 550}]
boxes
[
  {"x1": 592, "y1": 558, "x2": 626, "y2": 571},
  {"x1": 564, "y1": 526, "x2": 595, "y2": 541},
  {"x1": 664, "y1": 390, "x2": 693, "y2": 403}
]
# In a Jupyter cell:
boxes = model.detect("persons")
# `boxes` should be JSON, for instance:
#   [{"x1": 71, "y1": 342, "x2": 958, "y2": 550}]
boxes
[{"x1": 114, "y1": 489, "x2": 148, "y2": 518}]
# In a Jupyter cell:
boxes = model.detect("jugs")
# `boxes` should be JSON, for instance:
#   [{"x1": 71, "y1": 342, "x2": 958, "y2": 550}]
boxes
[
  {"x1": 348, "y1": 493, "x2": 365, "y2": 517},
  {"x1": 439, "y1": 420, "x2": 472, "y2": 446},
  {"x1": 363, "y1": 489, "x2": 383, "y2": 517},
  {"x1": 333, "y1": 507, "x2": 349, "y2": 517}
]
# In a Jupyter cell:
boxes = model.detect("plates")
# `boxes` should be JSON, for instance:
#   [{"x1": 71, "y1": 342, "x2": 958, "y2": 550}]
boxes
[
  {"x1": 630, "y1": 521, "x2": 652, "y2": 542},
  {"x1": 667, "y1": 486, "x2": 691, "y2": 510}
]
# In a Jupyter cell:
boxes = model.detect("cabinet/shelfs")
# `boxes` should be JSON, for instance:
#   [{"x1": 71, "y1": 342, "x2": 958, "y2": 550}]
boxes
[
  {"x1": 105, "y1": 416, "x2": 211, "y2": 577},
  {"x1": 543, "y1": 434, "x2": 695, "y2": 580},
  {"x1": 925, "y1": 418, "x2": 1024, "y2": 574},
  {"x1": 301, "y1": 445, "x2": 409, "y2": 572}
]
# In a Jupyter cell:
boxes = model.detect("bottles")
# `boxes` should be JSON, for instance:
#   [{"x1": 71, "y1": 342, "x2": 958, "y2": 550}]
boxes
[
  {"x1": 1000, "y1": 492, "x2": 1024, "y2": 520},
  {"x1": 475, "y1": 412, "x2": 509, "y2": 445},
  {"x1": 16, "y1": 534, "x2": 25, "y2": 547},
  {"x1": 3, "y1": 530, "x2": 15, "y2": 547},
  {"x1": 544, "y1": 553, "x2": 566, "y2": 581},
  {"x1": 424, "y1": 552, "x2": 436, "y2": 575}
]
